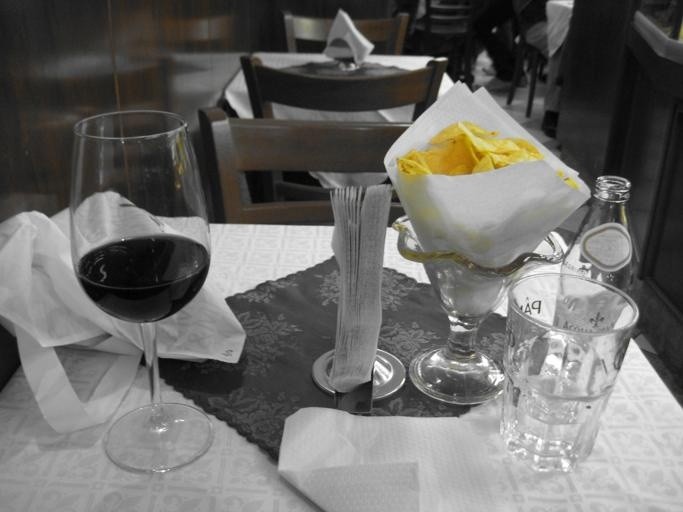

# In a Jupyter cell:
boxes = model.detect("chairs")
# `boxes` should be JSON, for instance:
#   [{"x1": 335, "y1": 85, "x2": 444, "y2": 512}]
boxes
[
  {"x1": 205, "y1": 102, "x2": 423, "y2": 223},
  {"x1": 242, "y1": 48, "x2": 447, "y2": 122},
  {"x1": 427, "y1": 0, "x2": 548, "y2": 119}
]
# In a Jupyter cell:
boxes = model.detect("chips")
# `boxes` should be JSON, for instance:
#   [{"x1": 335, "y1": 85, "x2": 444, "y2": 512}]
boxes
[{"x1": 396, "y1": 120, "x2": 579, "y2": 189}]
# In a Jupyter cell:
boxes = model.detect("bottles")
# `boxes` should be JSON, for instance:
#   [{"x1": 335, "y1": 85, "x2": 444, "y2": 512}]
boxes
[{"x1": 552, "y1": 174, "x2": 642, "y2": 337}]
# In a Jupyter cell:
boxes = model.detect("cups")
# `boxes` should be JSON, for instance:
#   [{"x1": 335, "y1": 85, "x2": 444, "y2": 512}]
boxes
[{"x1": 498, "y1": 271, "x2": 641, "y2": 477}]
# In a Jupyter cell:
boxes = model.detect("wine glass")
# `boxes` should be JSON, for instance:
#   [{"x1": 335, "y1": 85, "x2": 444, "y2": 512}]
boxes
[
  {"x1": 67, "y1": 109, "x2": 215, "y2": 473},
  {"x1": 390, "y1": 210, "x2": 568, "y2": 408}
]
